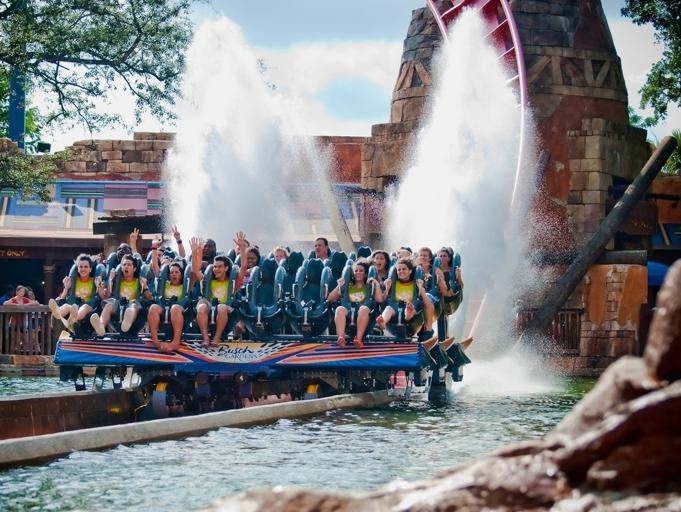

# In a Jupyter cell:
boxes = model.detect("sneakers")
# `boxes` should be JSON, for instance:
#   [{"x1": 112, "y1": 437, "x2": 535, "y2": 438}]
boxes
[
  {"x1": 119, "y1": 307, "x2": 136, "y2": 333},
  {"x1": 90, "y1": 313, "x2": 106, "y2": 337}
]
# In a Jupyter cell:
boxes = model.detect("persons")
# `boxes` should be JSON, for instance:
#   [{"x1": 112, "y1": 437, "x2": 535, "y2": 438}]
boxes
[
  {"x1": 1, "y1": 286, "x2": 40, "y2": 351},
  {"x1": 48, "y1": 225, "x2": 465, "y2": 353}
]
